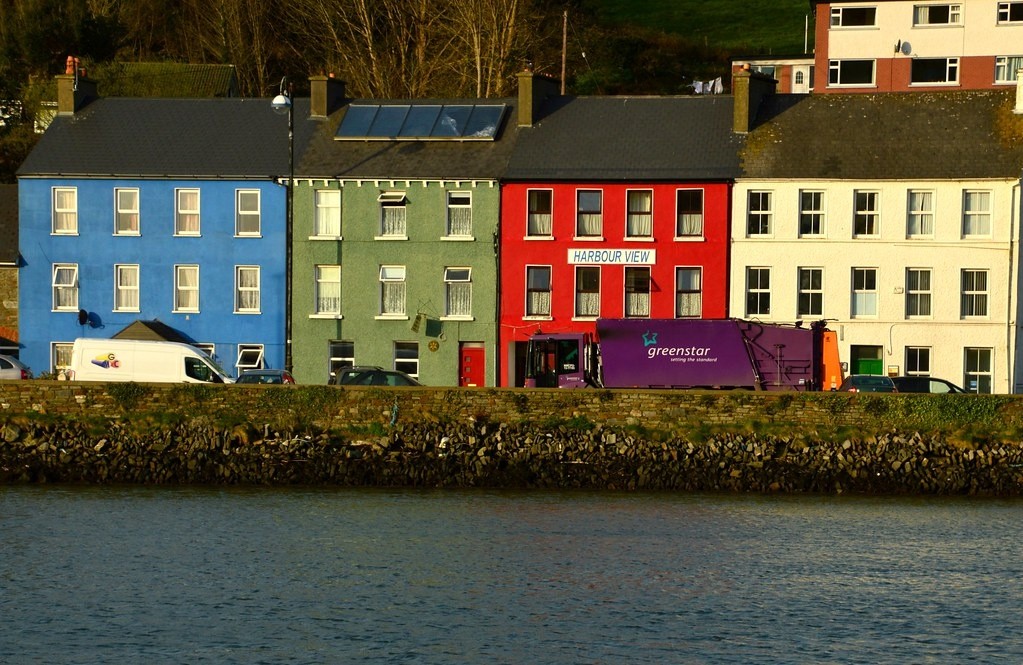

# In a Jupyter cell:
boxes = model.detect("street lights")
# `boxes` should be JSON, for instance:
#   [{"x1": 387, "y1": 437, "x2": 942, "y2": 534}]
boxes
[{"x1": 272, "y1": 90, "x2": 295, "y2": 373}]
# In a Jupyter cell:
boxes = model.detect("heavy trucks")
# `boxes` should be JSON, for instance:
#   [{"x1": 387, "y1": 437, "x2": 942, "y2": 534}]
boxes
[{"x1": 524, "y1": 315, "x2": 845, "y2": 390}]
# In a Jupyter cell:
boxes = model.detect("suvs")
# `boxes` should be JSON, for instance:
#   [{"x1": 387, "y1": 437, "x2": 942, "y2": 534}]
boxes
[{"x1": 328, "y1": 364, "x2": 425, "y2": 387}]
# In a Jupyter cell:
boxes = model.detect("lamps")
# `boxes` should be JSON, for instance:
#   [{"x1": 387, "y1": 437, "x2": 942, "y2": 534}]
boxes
[{"x1": 15, "y1": 251, "x2": 21, "y2": 266}]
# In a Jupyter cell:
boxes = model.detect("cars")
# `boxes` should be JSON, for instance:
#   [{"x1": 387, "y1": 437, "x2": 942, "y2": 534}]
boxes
[
  {"x1": 890, "y1": 376, "x2": 969, "y2": 395},
  {"x1": 235, "y1": 368, "x2": 295, "y2": 385},
  {"x1": 838, "y1": 373, "x2": 899, "y2": 396},
  {"x1": 0, "y1": 353, "x2": 34, "y2": 381}
]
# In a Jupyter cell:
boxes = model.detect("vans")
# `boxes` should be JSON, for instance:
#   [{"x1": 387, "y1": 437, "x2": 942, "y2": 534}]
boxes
[{"x1": 69, "y1": 337, "x2": 235, "y2": 384}]
